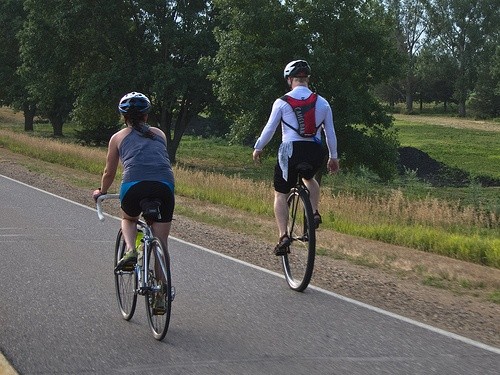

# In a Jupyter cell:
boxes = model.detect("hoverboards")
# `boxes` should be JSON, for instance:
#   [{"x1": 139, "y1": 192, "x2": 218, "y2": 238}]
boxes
[{"x1": 275, "y1": 163, "x2": 319, "y2": 292}]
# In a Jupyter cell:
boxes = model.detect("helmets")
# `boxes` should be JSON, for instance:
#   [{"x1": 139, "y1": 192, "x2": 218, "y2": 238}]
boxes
[
  {"x1": 119, "y1": 92, "x2": 150, "y2": 116},
  {"x1": 283, "y1": 60, "x2": 311, "y2": 78}
]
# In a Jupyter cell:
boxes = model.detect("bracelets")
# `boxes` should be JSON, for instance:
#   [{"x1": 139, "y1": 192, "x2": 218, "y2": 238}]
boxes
[{"x1": 98, "y1": 188, "x2": 107, "y2": 195}]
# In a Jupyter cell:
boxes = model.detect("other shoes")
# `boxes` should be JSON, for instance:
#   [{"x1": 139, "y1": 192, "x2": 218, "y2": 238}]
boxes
[
  {"x1": 116, "y1": 251, "x2": 137, "y2": 269},
  {"x1": 155, "y1": 299, "x2": 165, "y2": 314}
]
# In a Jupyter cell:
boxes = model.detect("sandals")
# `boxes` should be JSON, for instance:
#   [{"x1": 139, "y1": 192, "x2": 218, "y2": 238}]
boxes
[
  {"x1": 272, "y1": 235, "x2": 290, "y2": 255},
  {"x1": 313, "y1": 209, "x2": 322, "y2": 222}
]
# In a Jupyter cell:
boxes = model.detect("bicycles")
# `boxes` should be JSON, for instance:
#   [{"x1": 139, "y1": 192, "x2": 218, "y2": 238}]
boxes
[{"x1": 96, "y1": 194, "x2": 173, "y2": 341}]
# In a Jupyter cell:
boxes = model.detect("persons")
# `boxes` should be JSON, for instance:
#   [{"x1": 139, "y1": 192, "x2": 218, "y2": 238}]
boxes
[
  {"x1": 93, "y1": 92, "x2": 175, "y2": 315},
  {"x1": 253, "y1": 59, "x2": 339, "y2": 256}
]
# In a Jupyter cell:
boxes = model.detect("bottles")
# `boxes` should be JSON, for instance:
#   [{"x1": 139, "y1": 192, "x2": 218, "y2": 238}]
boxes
[
  {"x1": 137, "y1": 237, "x2": 145, "y2": 267},
  {"x1": 135, "y1": 229, "x2": 144, "y2": 250}
]
